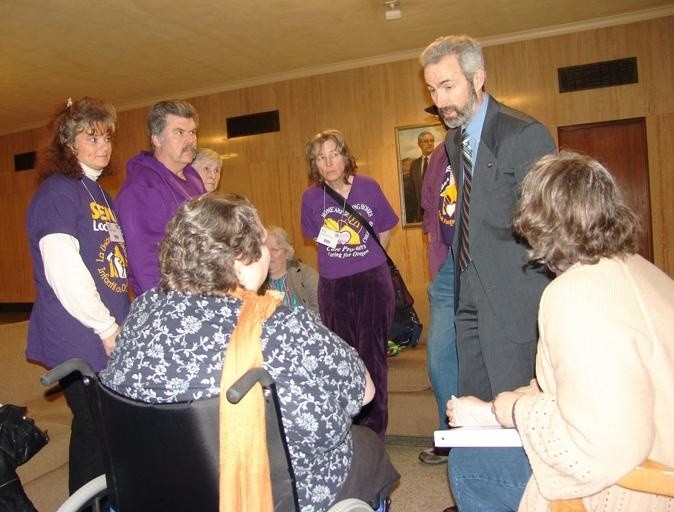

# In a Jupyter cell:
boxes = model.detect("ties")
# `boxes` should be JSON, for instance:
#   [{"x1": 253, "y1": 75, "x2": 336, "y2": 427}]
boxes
[
  {"x1": 458, "y1": 128, "x2": 474, "y2": 272},
  {"x1": 421, "y1": 156, "x2": 433, "y2": 179}
]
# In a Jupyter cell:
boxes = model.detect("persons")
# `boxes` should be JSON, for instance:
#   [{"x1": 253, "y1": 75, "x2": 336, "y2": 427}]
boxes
[
  {"x1": 191, "y1": 147, "x2": 222, "y2": 193},
  {"x1": 418, "y1": 139, "x2": 458, "y2": 465},
  {"x1": 416, "y1": 34, "x2": 556, "y2": 512},
  {"x1": 113, "y1": 100, "x2": 207, "y2": 297},
  {"x1": 444, "y1": 149, "x2": 672, "y2": 510},
  {"x1": 409, "y1": 129, "x2": 434, "y2": 224},
  {"x1": 24, "y1": 98, "x2": 129, "y2": 511},
  {"x1": 261, "y1": 223, "x2": 320, "y2": 316},
  {"x1": 98, "y1": 193, "x2": 402, "y2": 512},
  {"x1": 299, "y1": 129, "x2": 400, "y2": 443}
]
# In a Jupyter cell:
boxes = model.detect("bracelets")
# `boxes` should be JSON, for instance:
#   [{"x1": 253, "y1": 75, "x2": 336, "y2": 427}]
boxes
[{"x1": 491, "y1": 397, "x2": 501, "y2": 428}]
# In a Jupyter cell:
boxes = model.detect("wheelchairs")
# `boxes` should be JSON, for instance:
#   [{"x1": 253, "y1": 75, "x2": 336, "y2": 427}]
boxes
[{"x1": 42, "y1": 353, "x2": 400, "y2": 512}]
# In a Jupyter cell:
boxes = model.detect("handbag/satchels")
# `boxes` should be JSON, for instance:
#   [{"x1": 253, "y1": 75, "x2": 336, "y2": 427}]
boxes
[{"x1": 390, "y1": 267, "x2": 415, "y2": 309}]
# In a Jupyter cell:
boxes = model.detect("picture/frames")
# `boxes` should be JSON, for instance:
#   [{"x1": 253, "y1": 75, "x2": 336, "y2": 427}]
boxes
[{"x1": 391, "y1": 119, "x2": 444, "y2": 228}]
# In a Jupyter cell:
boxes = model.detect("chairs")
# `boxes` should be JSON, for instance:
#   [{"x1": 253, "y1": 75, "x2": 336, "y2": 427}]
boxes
[{"x1": 548, "y1": 458, "x2": 673, "y2": 512}]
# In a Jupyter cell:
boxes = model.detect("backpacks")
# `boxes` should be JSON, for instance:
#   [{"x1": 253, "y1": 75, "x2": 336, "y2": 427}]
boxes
[{"x1": 388, "y1": 304, "x2": 423, "y2": 349}]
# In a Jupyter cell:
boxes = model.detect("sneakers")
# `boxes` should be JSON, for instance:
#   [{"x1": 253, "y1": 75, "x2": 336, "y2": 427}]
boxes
[{"x1": 419, "y1": 448, "x2": 448, "y2": 466}]
